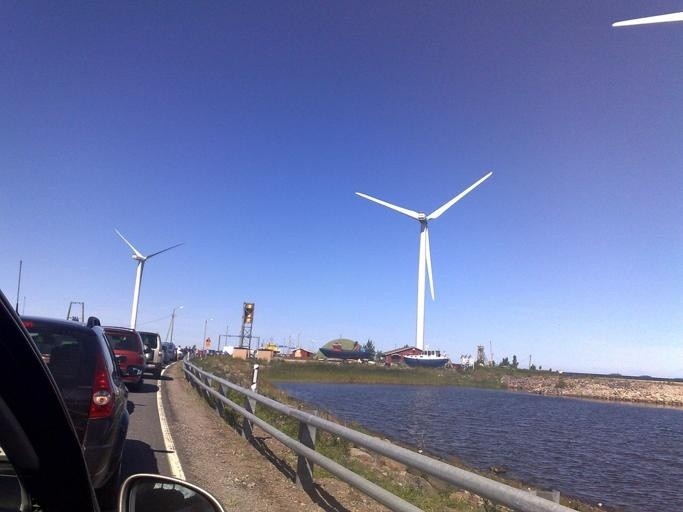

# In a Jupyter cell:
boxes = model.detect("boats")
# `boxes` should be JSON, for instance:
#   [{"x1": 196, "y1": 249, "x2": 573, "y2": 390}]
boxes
[
  {"x1": 319, "y1": 339, "x2": 374, "y2": 360},
  {"x1": 403, "y1": 345, "x2": 448, "y2": 368}
]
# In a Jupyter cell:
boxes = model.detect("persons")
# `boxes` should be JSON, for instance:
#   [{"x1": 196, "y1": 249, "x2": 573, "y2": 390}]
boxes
[{"x1": 177, "y1": 345, "x2": 197, "y2": 360}]
